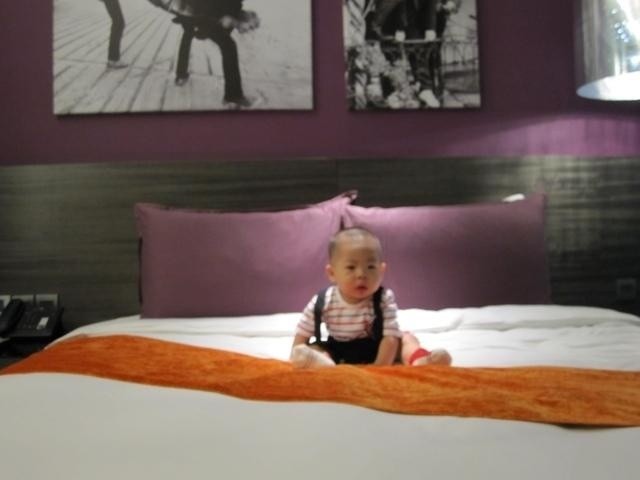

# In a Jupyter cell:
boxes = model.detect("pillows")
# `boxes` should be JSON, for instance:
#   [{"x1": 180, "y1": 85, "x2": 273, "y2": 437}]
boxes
[
  {"x1": 342, "y1": 194, "x2": 547, "y2": 311},
  {"x1": 135, "y1": 190, "x2": 358, "y2": 317}
]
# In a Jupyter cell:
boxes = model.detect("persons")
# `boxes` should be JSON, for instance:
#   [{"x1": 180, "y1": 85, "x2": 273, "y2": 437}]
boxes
[
  {"x1": 102, "y1": 0, "x2": 259, "y2": 107},
  {"x1": 291, "y1": 225, "x2": 452, "y2": 369},
  {"x1": 371, "y1": 0, "x2": 463, "y2": 109}
]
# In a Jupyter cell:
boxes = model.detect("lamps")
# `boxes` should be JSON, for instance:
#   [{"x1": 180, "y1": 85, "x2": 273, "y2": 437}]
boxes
[{"x1": 573, "y1": 0, "x2": 640, "y2": 101}]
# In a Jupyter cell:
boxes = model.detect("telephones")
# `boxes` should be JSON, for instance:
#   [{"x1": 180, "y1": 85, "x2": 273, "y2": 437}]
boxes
[{"x1": 0, "y1": 297, "x2": 64, "y2": 354}]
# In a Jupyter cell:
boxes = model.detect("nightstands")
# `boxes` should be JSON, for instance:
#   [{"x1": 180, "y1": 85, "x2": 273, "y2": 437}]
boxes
[{"x1": 0, "y1": 328, "x2": 71, "y2": 369}]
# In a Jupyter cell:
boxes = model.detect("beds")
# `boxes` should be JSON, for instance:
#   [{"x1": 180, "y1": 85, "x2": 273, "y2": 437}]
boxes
[{"x1": 0, "y1": 304, "x2": 640, "y2": 480}]
